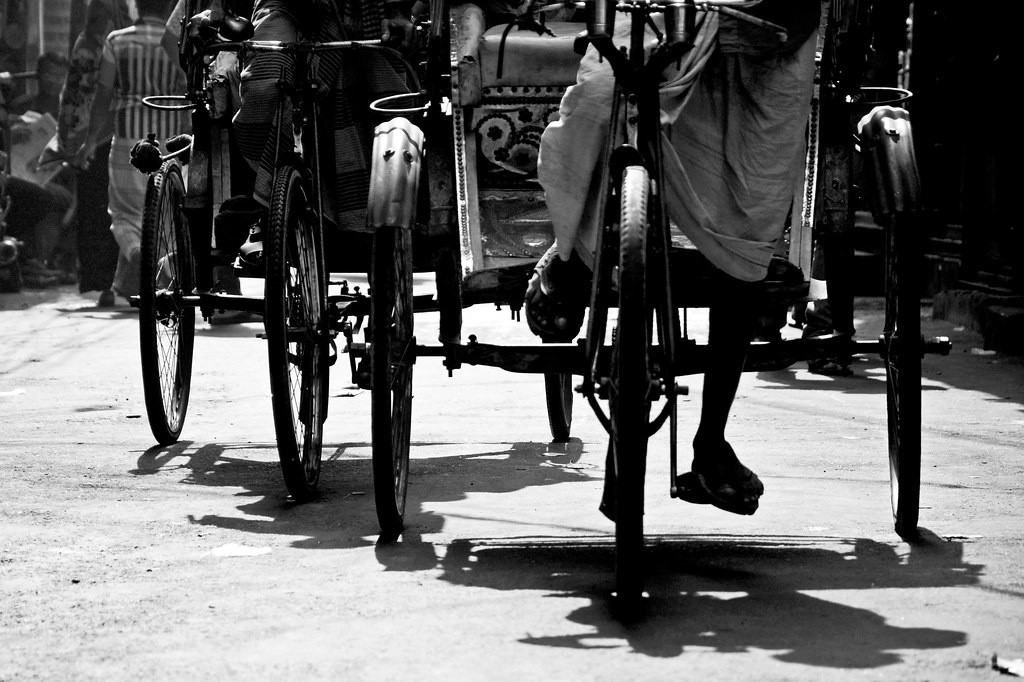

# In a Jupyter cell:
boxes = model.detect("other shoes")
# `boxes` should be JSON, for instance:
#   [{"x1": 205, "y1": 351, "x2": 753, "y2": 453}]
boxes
[
  {"x1": 218, "y1": 195, "x2": 252, "y2": 211},
  {"x1": 209, "y1": 308, "x2": 252, "y2": 324},
  {"x1": 98, "y1": 290, "x2": 115, "y2": 306}
]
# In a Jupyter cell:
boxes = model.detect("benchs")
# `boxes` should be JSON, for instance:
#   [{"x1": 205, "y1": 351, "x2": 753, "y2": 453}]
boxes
[{"x1": 453, "y1": 0, "x2": 596, "y2": 104}]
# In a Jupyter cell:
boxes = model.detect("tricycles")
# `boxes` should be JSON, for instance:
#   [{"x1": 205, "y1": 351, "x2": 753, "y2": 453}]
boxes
[
  {"x1": 127, "y1": 1, "x2": 576, "y2": 504},
  {"x1": 347, "y1": 0, "x2": 957, "y2": 631}
]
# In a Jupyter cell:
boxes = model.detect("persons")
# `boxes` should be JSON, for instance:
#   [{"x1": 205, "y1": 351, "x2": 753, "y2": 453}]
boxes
[
  {"x1": 48, "y1": 1, "x2": 122, "y2": 309},
  {"x1": 805, "y1": 290, "x2": 857, "y2": 377},
  {"x1": 1, "y1": 53, "x2": 83, "y2": 294},
  {"x1": 77, "y1": 0, "x2": 196, "y2": 309},
  {"x1": 516, "y1": 1, "x2": 825, "y2": 513},
  {"x1": 190, "y1": 0, "x2": 435, "y2": 393}
]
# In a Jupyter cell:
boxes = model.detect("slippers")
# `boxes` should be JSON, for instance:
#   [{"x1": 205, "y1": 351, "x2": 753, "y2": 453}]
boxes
[
  {"x1": 353, "y1": 318, "x2": 370, "y2": 387},
  {"x1": 808, "y1": 358, "x2": 854, "y2": 376},
  {"x1": 692, "y1": 441, "x2": 758, "y2": 515},
  {"x1": 241, "y1": 207, "x2": 266, "y2": 271},
  {"x1": 526, "y1": 243, "x2": 585, "y2": 341}
]
